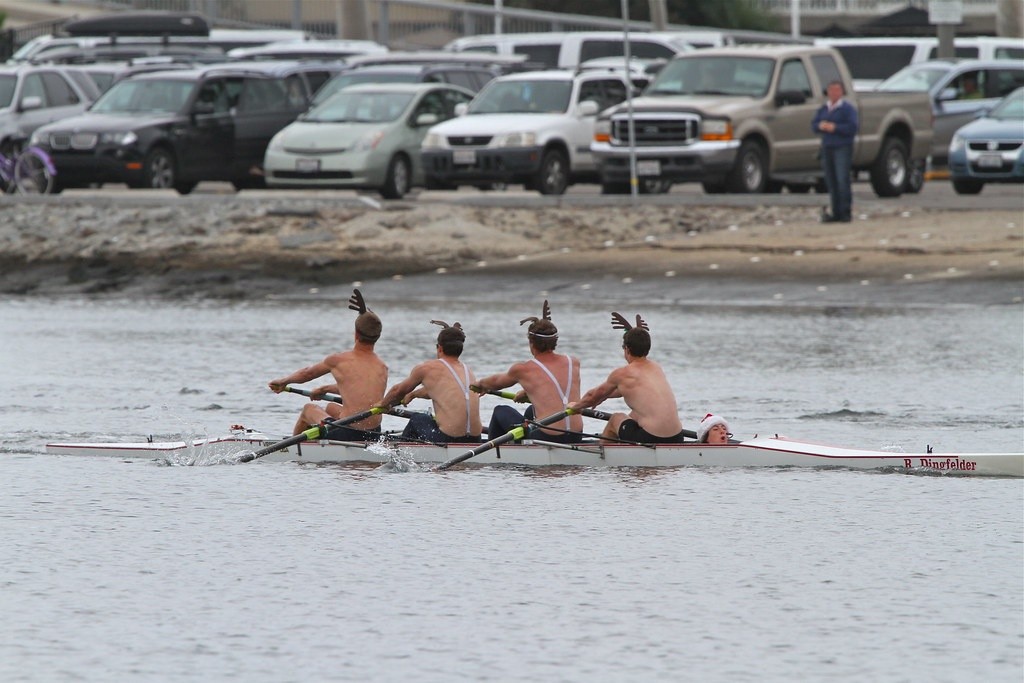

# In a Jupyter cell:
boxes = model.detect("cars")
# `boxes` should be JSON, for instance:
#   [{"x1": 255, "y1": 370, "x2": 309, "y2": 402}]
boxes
[
  {"x1": 874, "y1": 59, "x2": 1024, "y2": 169},
  {"x1": 0, "y1": 9, "x2": 1024, "y2": 161},
  {"x1": 255, "y1": 82, "x2": 484, "y2": 199},
  {"x1": 946, "y1": 84, "x2": 1024, "y2": 194}
]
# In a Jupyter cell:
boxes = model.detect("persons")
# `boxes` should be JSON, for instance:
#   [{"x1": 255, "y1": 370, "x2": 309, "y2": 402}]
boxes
[
  {"x1": 811, "y1": 81, "x2": 859, "y2": 222},
  {"x1": 565, "y1": 329, "x2": 683, "y2": 443},
  {"x1": 269, "y1": 312, "x2": 388, "y2": 440},
  {"x1": 697, "y1": 413, "x2": 728, "y2": 444},
  {"x1": 372, "y1": 327, "x2": 482, "y2": 444},
  {"x1": 471, "y1": 319, "x2": 583, "y2": 444}
]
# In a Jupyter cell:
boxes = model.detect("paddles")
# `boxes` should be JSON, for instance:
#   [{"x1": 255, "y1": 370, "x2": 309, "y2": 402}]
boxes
[
  {"x1": 238, "y1": 396, "x2": 402, "y2": 463},
  {"x1": 279, "y1": 382, "x2": 434, "y2": 420},
  {"x1": 427, "y1": 407, "x2": 576, "y2": 472},
  {"x1": 468, "y1": 382, "x2": 705, "y2": 439}
]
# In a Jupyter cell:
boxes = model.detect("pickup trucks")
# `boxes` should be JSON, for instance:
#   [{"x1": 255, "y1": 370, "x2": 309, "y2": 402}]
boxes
[{"x1": 589, "y1": 42, "x2": 936, "y2": 196}]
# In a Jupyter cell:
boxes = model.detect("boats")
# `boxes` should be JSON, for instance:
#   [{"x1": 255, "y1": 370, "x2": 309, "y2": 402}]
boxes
[{"x1": 44, "y1": 426, "x2": 1024, "y2": 480}]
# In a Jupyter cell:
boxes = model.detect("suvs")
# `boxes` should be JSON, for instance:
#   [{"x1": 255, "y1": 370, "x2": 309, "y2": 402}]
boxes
[
  {"x1": 415, "y1": 63, "x2": 655, "y2": 198},
  {"x1": 28, "y1": 61, "x2": 311, "y2": 190},
  {"x1": 0, "y1": 63, "x2": 110, "y2": 193}
]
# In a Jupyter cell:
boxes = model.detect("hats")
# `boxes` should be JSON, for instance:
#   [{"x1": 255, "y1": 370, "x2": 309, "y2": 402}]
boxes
[{"x1": 696, "y1": 413, "x2": 728, "y2": 442}]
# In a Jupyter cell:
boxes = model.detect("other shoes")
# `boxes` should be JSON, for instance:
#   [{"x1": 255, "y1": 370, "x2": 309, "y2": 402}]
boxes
[{"x1": 830, "y1": 212, "x2": 851, "y2": 223}]
